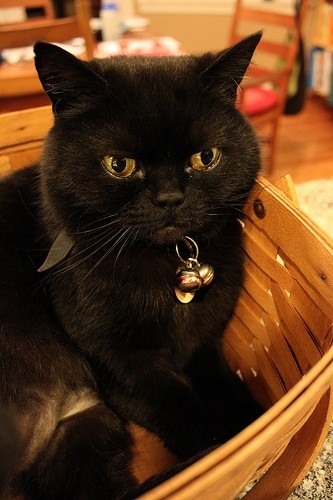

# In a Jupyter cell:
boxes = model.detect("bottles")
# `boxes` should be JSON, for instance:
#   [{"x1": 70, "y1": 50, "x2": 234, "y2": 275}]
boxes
[{"x1": 100, "y1": 3, "x2": 121, "y2": 42}]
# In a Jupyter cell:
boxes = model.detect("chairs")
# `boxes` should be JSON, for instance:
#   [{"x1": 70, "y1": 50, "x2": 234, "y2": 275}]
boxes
[{"x1": 227, "y1": 0, "x2": 308, "y2": 179}]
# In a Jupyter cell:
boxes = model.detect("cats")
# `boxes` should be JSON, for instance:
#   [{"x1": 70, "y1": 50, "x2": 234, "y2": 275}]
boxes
[{"x1": 0, "y1": 29, "x2": 267, "y2": 500}]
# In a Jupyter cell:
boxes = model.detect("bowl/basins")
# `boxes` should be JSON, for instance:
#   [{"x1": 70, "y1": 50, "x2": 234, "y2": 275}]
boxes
[{"x1": 126, "y1": 18, "x2": 148, "y2": 32}]
